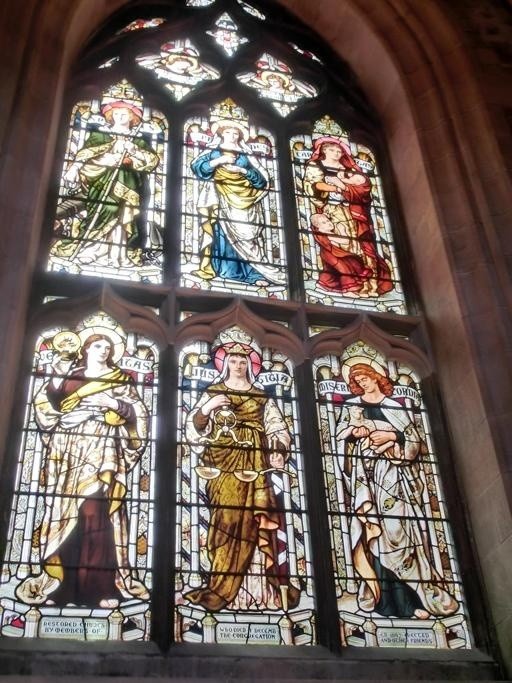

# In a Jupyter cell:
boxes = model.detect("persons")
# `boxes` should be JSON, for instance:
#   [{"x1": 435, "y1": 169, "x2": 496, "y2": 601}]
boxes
[
  {"x1": 57, "y1": 89, "x2": 164, "y2": 271},
  {"x1": 183, "y1": 124, "x2": 288, "y2": 286},
  {"x1": 188, "y1": 326, "x2": 304, "y2": 614},
  {"x1": 330, "y1": 337, "x2": 461, "y2": 620},
  {"x1": 301, "y1": 134, "x2": 394, "y2": 297},
  {"x1": 32, "y1": 323, "x2": 149, "y2": 609},
  {"x1": 153, "y1": 43, "x2": 207, "y2": 98},
  {"x1": 255, "y1": 64, "x2": 308, "y2": 118}
]
